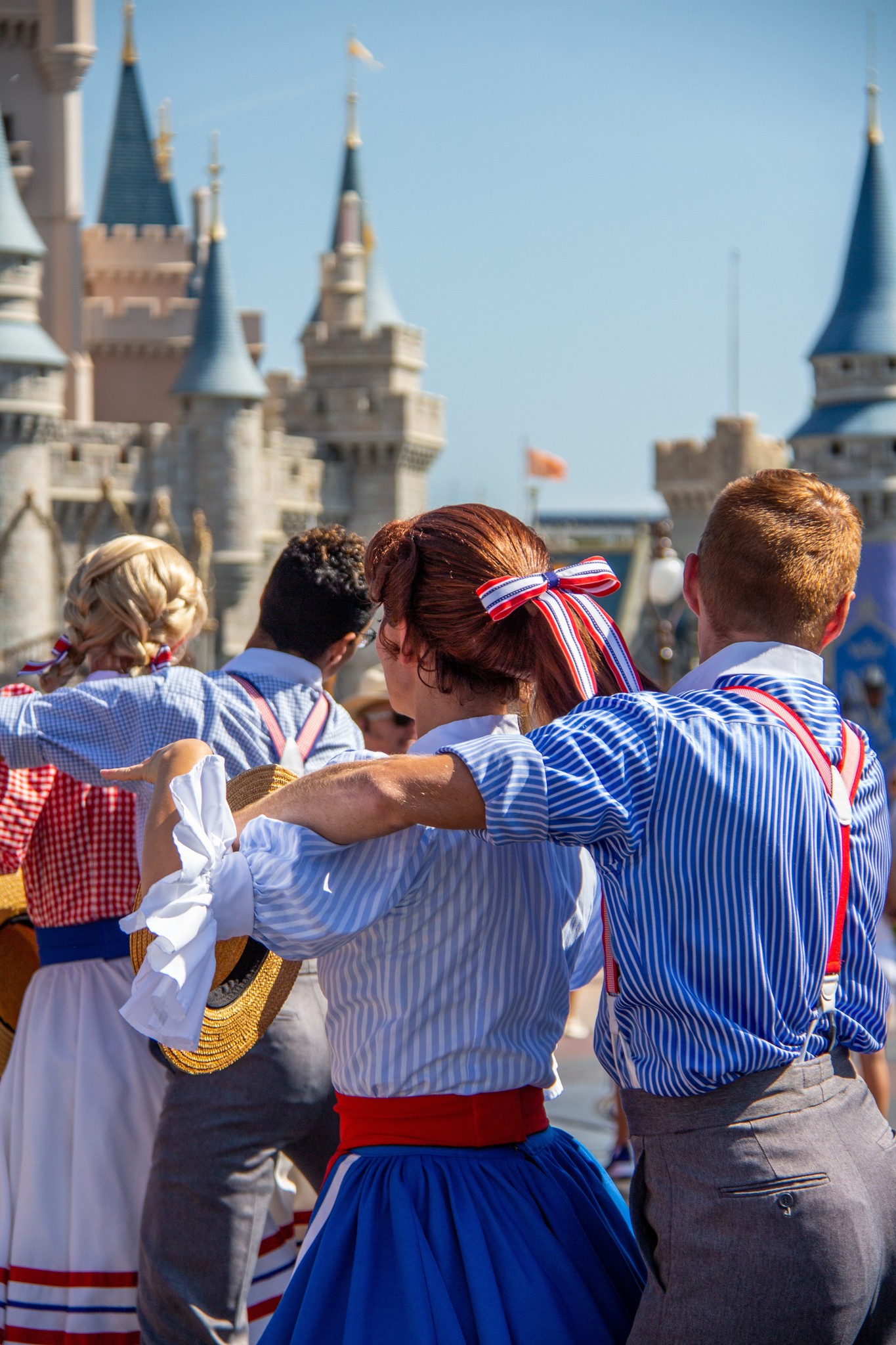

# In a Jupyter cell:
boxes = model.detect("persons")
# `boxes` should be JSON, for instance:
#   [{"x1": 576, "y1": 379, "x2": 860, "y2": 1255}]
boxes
[
  {"x1": 220, "y1": 467, "x2": 895, "y2": 1345},
  {"x1": 98, "y1": 502, "x2": 661, "y2": 1343},
  {"x1": 1, "y1": 530, "x2": 315, "y2": 1345},
  {"x1": 839, "y1": 666, "x2": 896, "y2": 919},
  {"x1": 341, "y1": 690, "x2": 417, "y2": 763},
  {"x1": 0, "y1": 519, "x2": 383, "y2": 1345}
]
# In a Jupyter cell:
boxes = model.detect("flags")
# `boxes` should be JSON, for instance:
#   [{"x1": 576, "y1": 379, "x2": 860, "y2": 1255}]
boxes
[{"x1": 527, "y1": 447, "x2": 568, "y2": 480}]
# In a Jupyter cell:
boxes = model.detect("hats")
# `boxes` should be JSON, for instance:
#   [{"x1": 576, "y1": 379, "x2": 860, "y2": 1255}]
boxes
[
  {"x1": 128, "y1": 762, "x2": 304, "y2": 1074},
  {"x1": 0, "y1": 869, "x2": 39, "y2": 1077},
  {"x1": 338, "y1": 663, "x2": 390, "y2": 713}
]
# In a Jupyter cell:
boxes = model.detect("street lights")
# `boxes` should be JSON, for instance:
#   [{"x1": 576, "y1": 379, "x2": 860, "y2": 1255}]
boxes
[{"x1": 639, "y1": 516, "x2": 688, "y2": 690}]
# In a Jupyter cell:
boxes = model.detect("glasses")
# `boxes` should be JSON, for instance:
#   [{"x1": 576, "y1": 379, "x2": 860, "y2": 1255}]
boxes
[{"x1": 368, "y1": 710, "x2": 413, "y2": 727}]
